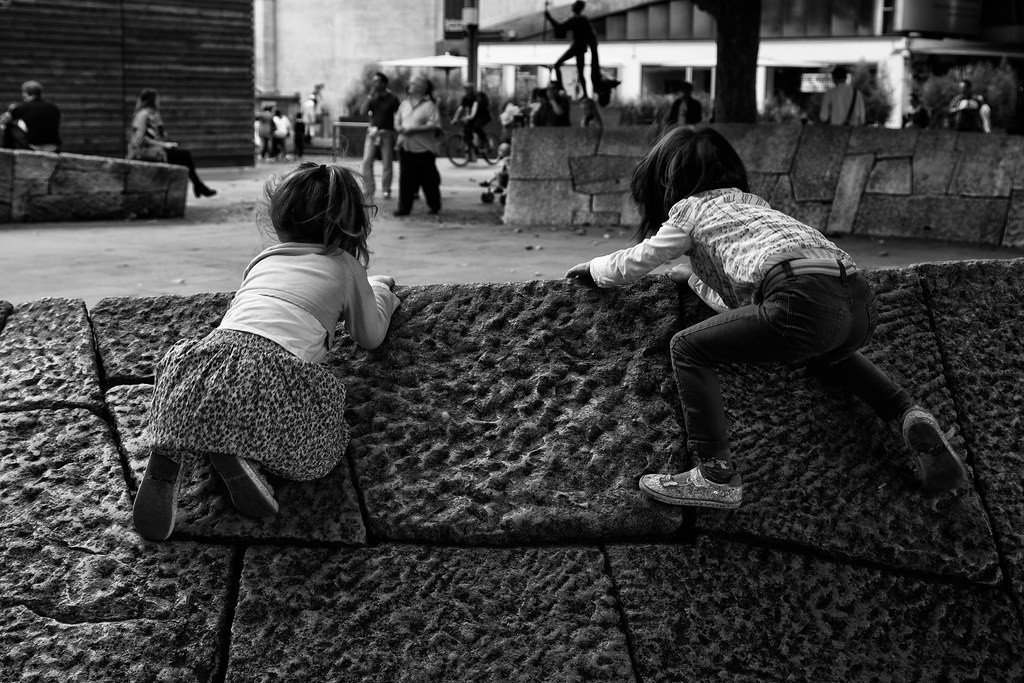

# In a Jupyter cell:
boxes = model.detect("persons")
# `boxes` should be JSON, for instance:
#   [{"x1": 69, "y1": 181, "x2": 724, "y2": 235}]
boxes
[
  {"x1": 901, "y1": 92, "x2": 930, "y2": 129},
  {"x1": 820, "y1": 64, "x2": 866, "y2": 127},
  {"x1": 132, "y1": 162, "x2": 403, "y2": 542},
  {"x1": 565, "y1": 127, "x2": 964, "y2": 509},
  {"x1": 254, "y1": 95, "x2": 317, "y2": 161},
  {"x1": 359, "y1": 71, "x2": 445, "y2": 218},
  {"x1": 126, "y1": 89, "x2": 216, "y2": 198},
  {"x1": 669, "y1": 82, "x2": 702, "y2": 125},
  {"x1": 543, "y1": 1, "x2": 603, "y2": 99},
  {"x1": 450, "y1": 82, "x2": 492, "y2": 153},
  {"x1": 0, "y1": 80, "x2": 62, "y2": 153},
  {"x1": 947, "y1": 79, "x2": 991, "y2": 134},
  {"x1": 498, "y1": 83, "x2": 599, "y2": 144}
]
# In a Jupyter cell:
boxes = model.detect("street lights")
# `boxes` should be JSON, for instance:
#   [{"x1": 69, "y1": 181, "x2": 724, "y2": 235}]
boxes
[{"x1": 461, "y1": 6, "x2": 480, "y2": 85}]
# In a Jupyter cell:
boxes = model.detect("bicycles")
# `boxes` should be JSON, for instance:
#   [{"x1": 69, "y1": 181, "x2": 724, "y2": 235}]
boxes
[{"x1": 448, "y1": 117, "x2": 502, "y2": 168}]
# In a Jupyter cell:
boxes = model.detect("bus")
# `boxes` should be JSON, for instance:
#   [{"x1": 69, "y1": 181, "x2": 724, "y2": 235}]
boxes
[{"x1": 478, "y1": 30, "x2": 1024, "y2": 134}]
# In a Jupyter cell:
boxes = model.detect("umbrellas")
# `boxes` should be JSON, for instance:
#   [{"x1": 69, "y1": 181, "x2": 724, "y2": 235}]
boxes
[{"x1": 376, "y1": 52, "x2": 502, "y2": 70}]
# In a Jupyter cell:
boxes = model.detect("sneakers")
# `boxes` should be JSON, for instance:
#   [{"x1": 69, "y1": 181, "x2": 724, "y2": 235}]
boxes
[
  {"x1": 638, "y1": 461, "x2": 743, "y2": 509},
  {"x1": 903, "y1": 409, "x2": 966, "y2": 492}
]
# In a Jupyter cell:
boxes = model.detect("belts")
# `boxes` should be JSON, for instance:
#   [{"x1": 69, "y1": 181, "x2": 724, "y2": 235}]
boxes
[{"x1": 760, "y1": 258, "x2": 857, "y2": 294}]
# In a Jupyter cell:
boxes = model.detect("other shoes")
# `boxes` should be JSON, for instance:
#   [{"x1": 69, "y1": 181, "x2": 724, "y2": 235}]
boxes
[
  {"x1": 383, "y1": 190, "x2": 390, "y2": 199},
  {"x1": 210, "y1": 448, "x2": 278, "y2": 516},
  {"x1": 428, "y1": 204, "x2": 440, "y2": 214},
  {"x1": 134, "y1": 453, "x2": 184, "y2": 542},
  {"x1": 392, "y1": 209, "x2": 408, "y2": 216}
]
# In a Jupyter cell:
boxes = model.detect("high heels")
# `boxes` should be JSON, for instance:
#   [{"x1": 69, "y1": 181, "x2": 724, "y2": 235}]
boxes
[{"x1": 194, "y1": 184, "x2": 217, "y2": 198}]
